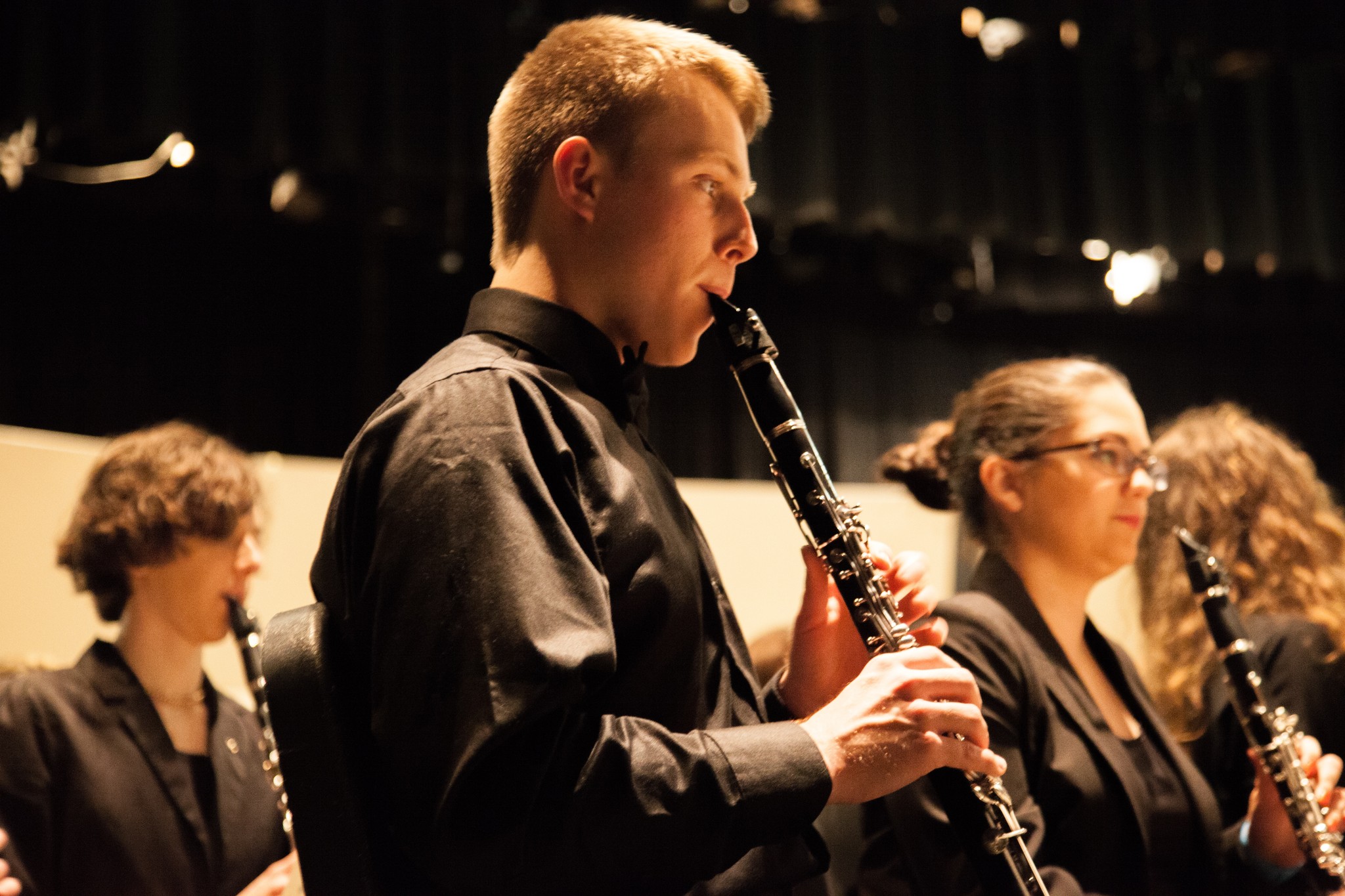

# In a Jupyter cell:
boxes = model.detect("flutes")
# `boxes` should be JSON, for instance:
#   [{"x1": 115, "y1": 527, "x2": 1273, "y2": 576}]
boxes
[
  {"x1": 226, "y1": 594, "x2": 301, "y2": 848},
  {"x1": 1170, "y1": 525, "x2": 1345, "y2": 887},
  {"x1": 707, "y1": 289, "x2": 1049, "y2": 896}
]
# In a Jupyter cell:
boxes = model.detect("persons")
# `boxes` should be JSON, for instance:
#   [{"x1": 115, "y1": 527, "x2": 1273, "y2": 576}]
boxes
[
  {"x1": 865, "y1": 355, "x2": 1345, "y2": 896},
  {"x1": 0, "y1": 423, "x2": 308, "y2": 896},
  {"x1": 311, "y1": 15, "x2": 1008, "y2": 896},
  {"x1": 1132, "y1": 398, "x2": 1345, "y2": 835},
  {"x1": 0, "y1": 827, "x2": 22, "y2": 895}
]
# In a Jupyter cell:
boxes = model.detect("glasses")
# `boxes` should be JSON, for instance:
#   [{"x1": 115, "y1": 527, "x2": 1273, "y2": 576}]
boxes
[{"x1": 1008, "y1": 432, "x2": 1170, "y2": 492}]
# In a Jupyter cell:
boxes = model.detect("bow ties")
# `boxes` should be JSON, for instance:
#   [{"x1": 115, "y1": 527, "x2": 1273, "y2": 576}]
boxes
[{"x1": 619, "y1": 340, "x2": 650, "y2": 440}]
